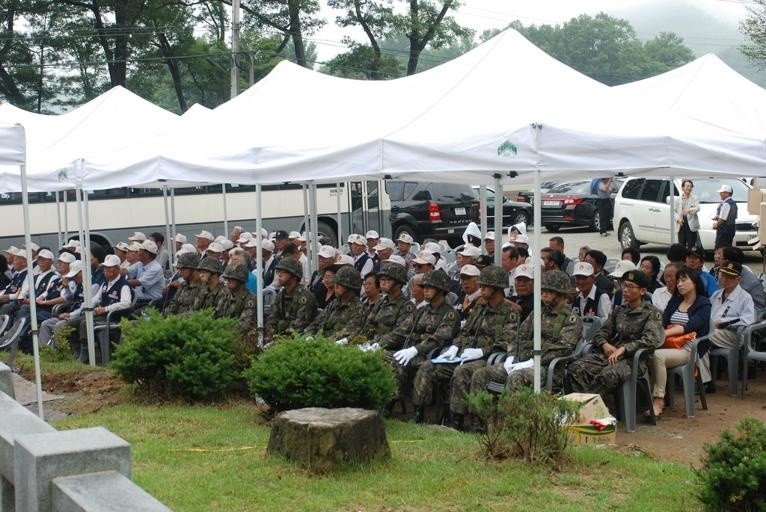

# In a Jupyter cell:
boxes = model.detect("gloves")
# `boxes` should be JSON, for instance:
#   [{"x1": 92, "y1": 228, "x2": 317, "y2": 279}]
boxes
[
  {"x1": 504, "y1": 356, "x2": 533, "y2": 374},
  {"x1": 438, "y1": 346, "x2": 483, "y2": 362},
  {"x1": 393, "y1": 346, "x2": 417, "y2": 366}
]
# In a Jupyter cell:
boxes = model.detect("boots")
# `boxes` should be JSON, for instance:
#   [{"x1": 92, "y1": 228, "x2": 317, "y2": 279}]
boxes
[
  {"x1": 448, "y1": 415, "x2": 483, "y2": 433},
  {"x1": 413, "y1": 406, "x2": 424, "y2": 423}
]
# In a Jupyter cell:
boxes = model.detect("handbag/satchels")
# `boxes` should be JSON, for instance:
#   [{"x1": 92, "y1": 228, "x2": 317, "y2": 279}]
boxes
[{"x1": 664, "y1": 324, "x2": 697, "y2": 348}]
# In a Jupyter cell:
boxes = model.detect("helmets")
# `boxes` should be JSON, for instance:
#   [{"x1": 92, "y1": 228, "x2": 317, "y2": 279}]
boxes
[
  {"x1": 541, "y1": 270, "x2": 571, "y2": 293},
  {"x1": 177, "y1": 252, "x2": 248, "y2": 280},
  {"x1": 275, "y1": 257, "x2": 302, "y2": 281},
  {"x1": 477, "y1": 265, "x2": 509, "y2": 288},
  {"x1": 376, "y1": 263, "x2": 407, "y2": 284},
  {"x1": 419, "y1": 271, "x2": 450, "y2": 294},
  {"x1": 333, "y1": 266, "x2": 361, "y2": 289}
]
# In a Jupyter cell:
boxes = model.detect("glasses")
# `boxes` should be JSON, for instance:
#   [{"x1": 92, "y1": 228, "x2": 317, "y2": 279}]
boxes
[{"x1": 621, "y1": 282, "x2": 634, "y2": 289}]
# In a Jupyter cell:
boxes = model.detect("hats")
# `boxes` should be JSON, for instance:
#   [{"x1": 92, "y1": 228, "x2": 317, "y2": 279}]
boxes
[
  {"x1": 101, "y1": 232, "x2": 159, "y2": 266},
  {"x1": 513, "y1": 234, "x2": 529, "y2": 245},
  {"x1": 687, "y1": 245, "x2": 705, "y2": 263},
  {"x1": 573, "y1": 260, "x2": 649, "y2": 287},
  {"x1": 171, "y1": 226, "x2": 440, "y2": 266},
  {"x1": 459, "y1": 245, "x2": 481, "y2": 275},
  {"x1": 513, "y1": 264, "x2": 534, "y2": 280},
  {"x1": 484, "y1": 231, "x2": 495, "y2": 240},
  {"x1": 3, "y1": 240, "x2": 81, "y2": 278},
  {"x1": 720, "y1": 260, "x2": 742, "y2": 277},
  {"x1": 718, "y1": 185, "x2": 732, "y2": 192}
]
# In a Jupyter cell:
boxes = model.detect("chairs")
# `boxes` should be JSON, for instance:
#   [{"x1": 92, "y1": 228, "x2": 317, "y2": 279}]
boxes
[
  {"x1": 0, "y1": 285, "x2": 140, "y2": 367},
  {"x1": 741, "y1": 264, "x2": 755, "y2": 275},
  {"x1": 427, "y1": 310, "x2": 765, "y2": 434},
  {"x1": 571, "y1": 256, "x2": 622, "y2": 273}
]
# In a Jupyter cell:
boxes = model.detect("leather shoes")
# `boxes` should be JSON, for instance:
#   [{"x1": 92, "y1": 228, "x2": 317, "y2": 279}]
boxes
[{"x1": 696, "y1": 384, "x2": 715, "y2": 394}]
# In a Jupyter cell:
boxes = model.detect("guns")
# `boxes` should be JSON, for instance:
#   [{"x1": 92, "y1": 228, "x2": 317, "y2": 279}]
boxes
[
  {"x1": 379, "y1": 308, "x2": 425, "y2": 418},
  {"x1": 439, "y1": 316, "x2": 486, "y2": 425},
  {"x1": 486, "y1": 311, "x2": 522, "y2": 394}
]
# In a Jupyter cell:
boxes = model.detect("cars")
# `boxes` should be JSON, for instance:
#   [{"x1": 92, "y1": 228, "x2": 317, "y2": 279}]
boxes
[
  {"x1": 539, "y1": 178, "x2": 622, "y2": 233},
  {"x1": 516, "y1": 190, "x2": 533, "y2": 207}
]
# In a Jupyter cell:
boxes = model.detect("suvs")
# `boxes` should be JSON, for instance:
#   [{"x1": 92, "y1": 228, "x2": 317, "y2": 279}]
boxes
[
  {"x1": 471, "y1": 186, "x2": 532, "y2": 232},
  {"x1": 350, "y1": 177, "x2": 478, "y2": 244}
]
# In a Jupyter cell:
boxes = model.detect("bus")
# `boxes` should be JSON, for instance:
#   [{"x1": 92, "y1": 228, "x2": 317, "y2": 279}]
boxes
[{"x1": 0, "y1": 174, "x2": 392, "y2": 262}]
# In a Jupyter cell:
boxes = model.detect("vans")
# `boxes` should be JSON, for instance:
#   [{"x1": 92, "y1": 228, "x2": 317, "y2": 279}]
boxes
[{"x1": 610, "y1": 173, "x2": 765, "y2": 258}]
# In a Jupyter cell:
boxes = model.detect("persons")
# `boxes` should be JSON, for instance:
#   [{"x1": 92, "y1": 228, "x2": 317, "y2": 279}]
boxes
[
  {"x1": 673, "y1": 181, "x2": 700, "y2": 250},
  {"x1": 712, "y1": 185, "x2": 739, "y2": 250},
  {"x1": 598, "y1": 177, "x2": 615, "y2": 238}
]
